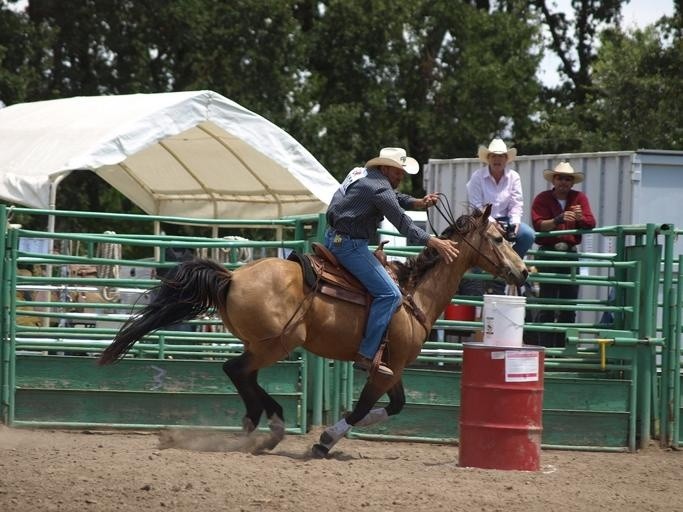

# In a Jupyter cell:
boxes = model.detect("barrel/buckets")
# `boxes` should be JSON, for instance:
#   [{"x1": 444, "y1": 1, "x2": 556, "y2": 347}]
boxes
[
  {"x1": 459, "y1": 343, "x2": 544, "y2": 471},
  {"x1": 483, "y1": 295, "x2": 527, "y2": 347},
  {"x1": 444, "y1": 303, "x2": 475, "y2": 335}
]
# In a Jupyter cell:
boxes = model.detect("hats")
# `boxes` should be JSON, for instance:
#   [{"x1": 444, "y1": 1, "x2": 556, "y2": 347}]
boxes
[
  {"x1": 475, "y1": 138, "x2": 518, "y2": 165},
  {"x1": 541, "y1": 160, "x2": 585, "y2": 185},
  {"x1": 364, "y1": 146, "x2": 419, "y2": 176}
]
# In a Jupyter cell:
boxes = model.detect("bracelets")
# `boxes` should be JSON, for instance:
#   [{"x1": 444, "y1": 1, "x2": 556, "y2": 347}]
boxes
[{"x1": 554, "y1": 216, "x2": 564, "y2": 225}]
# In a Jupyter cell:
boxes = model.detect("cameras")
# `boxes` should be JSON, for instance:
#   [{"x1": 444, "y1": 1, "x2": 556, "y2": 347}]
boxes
[{"x1": 500, "y1": 223, "x2": 518, "y2": 242}]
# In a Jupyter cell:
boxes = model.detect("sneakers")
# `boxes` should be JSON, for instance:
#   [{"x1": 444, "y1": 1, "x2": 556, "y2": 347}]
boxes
[{"x1": 351, "y1": 352, "x2": 394, "y2": 375}]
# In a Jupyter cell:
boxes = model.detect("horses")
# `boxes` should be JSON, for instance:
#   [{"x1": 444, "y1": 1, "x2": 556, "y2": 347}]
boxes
[{"x1": 95, "y1": 202, "x2": 528, "y2": 459}]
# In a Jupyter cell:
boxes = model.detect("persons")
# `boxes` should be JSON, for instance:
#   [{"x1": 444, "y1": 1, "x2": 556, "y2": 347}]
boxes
[
  {"x1": 530, "y1": 161, "x2": 596, "y2": 359},
  {"x1": 324, "y1": 145, "x2": 460, "y2": 376},
  {"x1": 465, "y1": 136, "x2": 536, "y2": 260}
]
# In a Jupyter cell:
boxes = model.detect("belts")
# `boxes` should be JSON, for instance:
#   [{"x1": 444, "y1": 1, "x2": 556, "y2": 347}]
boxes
[
  {"x1": 552, "y1": 242, "x2": 577, "y2": 253},
  {"x1": 334, "y1": 230, "x2": 368, "y2": 239}
]
